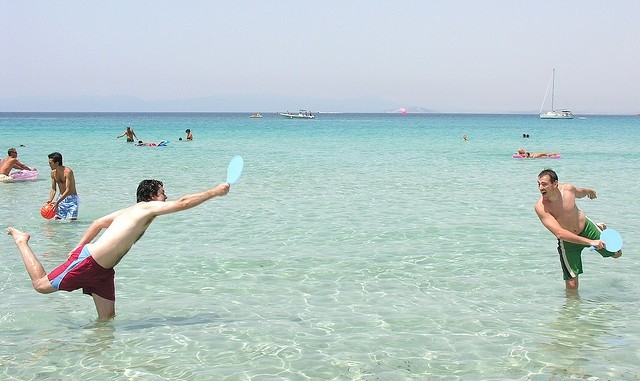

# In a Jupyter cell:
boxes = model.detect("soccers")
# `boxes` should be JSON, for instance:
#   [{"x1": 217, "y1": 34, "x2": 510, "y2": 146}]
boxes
[{"x1": 41, "y1": 205, "x2": 55, "y2": 218}]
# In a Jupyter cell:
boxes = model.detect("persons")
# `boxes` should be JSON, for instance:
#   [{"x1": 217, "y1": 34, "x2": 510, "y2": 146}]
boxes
[
  {"x1": 463, "y1": 135, "x2": 468, "y2": 142},
  {"x1": 185, "y1": 129, "x2": 193, "y2": 141},
  {"x1": 0, "y1": 148, "x2": 37, "y2": 177},
  {"x1": 527, "y1": 134, "x2": 529, "y2": 138},
  {"x1": 523, "y1": 133, "x2": 526, "y2": 137},
  {"x1": 7, "y1": 180, "x2": 230, "y2": 320},
  {"x1": 534, "y1": 170, "x2": 622, "y2": 291},
  {"x1": 179, "y1": 137, "x2": 183, "y2": 142},
  {"x1": 47, "y1": 153, "x2": 79, "y2": 224},
  {"x1": 515, "y1": 149, "x2": 559, "y2": 159},
  {"x1": 116, "y1": 127, "x2": 138, "y2": 142},
  {"x1": 135, "y1": 140, "x2": 165, "y2": 147}
]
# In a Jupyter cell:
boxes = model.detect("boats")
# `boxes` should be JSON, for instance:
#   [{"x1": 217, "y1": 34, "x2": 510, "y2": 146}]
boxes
[
  {"x1": 249, "y1": 113, "x2": 262, "y2": 118},
  {"x1": 281, "y1": 110, "x2": 315, "y2": 118}
]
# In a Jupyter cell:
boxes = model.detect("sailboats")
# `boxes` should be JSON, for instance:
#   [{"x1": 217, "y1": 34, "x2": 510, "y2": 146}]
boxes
[{"x1": 540, "y1": 68, "x2": 574, "y2": 118}]
